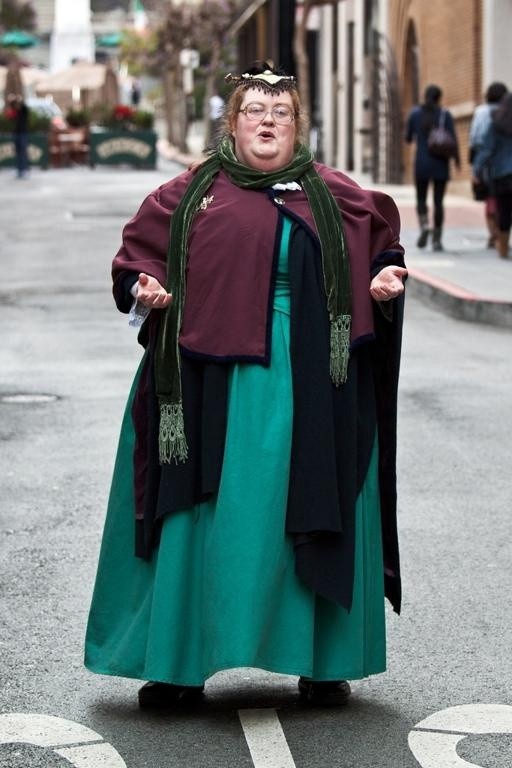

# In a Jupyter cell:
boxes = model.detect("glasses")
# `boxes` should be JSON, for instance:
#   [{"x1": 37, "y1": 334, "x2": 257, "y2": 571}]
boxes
[{"x1": 235, "y1": 102, "x2": 298, "y2": 127}]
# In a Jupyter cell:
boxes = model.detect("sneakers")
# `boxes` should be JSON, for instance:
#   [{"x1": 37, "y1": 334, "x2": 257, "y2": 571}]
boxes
[
  {"x1": 298, "y1": 677, "x2": 352, "y2": 709},
  {"x1": 486, "y1": 236, "x2": 509, "y2": 257},
  {"x1": 418, "y1": 228, "x2": 431, "y2": 246},
  {"x1": 431, "y1": 240, "x2": 442, "y2": 252},
  {"x1": 135, "y1": 681, "x2": 207, "y2": 712}
]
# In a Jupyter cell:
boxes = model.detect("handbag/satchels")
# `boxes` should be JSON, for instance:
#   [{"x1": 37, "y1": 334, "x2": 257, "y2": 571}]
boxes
[{"x1": 426, "y1": 107, "x2": 456, "y2": 154}]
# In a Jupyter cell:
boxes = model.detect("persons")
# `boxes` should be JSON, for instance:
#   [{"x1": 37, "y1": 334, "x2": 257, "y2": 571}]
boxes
[
  {"x1": 12, "y1": 93, "x2": 33, "y2": 177},
  {"x1": 472, "y1": 93, "x2": 511, "y2": 258},
  {"x1": 4, "y1": 90, "x2": 20, "y2": 129},
  {"x1": 405, "y1": 83, "x2": 464, "y2": 252},
  {"x1": 467, "y1": 81, "x2": 505, "y2": 247},
  {"x1": 85, "y1": 62, "x2": 406, "y2": 712},
  {"x1": 208, "y1": 85, "x2": 226, "y2": 147}
]
